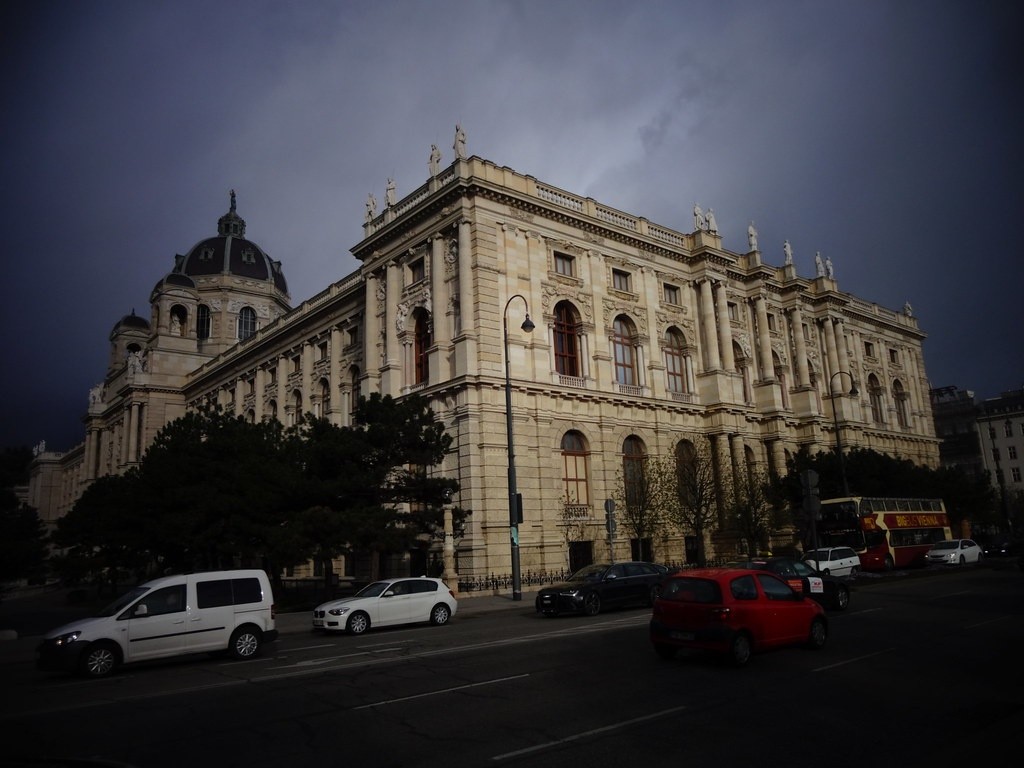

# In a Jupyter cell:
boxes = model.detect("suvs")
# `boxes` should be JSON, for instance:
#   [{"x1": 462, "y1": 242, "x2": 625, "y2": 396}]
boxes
[{"x1": 801, "y1": 546, "x2": 860, "y2": 583}]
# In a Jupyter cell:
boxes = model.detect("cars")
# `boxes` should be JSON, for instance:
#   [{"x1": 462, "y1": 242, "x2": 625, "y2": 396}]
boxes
[
  {"x1": 988, "y1": 535, "x2": 1024, "y2": 557},
  {"x1": 925, "y1": 538, "x2": 983, "y2": 568},
  {"x1": 313, "y1": 575, "x2": 459, "y2": 634},
  {"x1": 721, "y1": 556, "x2": 850, "y2": 612},
  {"x1": 536, "y1": 561, "x2": 670, "y2": 616},
  {"x1": 650, "y1": 568, "x2": 827, "y2": 668}
]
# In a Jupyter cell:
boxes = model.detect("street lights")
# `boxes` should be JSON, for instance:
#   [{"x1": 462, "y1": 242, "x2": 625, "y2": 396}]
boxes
[
  {"x1": 986, "y1": 403, "x2": 1012, "y2": 540},
  {"x1": 503, "y1": 294, "x2": 535, "y2": 601},
  {"x1": 830, "y1": 372, "x2": 858, "y2": 495}
]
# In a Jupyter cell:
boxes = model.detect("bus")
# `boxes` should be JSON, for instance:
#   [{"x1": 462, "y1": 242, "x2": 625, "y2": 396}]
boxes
[{"x1": 820, "y1": 498, "x2": 952, "y2": 573}]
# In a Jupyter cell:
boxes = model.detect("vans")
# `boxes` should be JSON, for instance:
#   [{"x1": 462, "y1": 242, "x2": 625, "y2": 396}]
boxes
[{"x1": 41, "y1": 570, "x2": 277, "y2": 680}]
